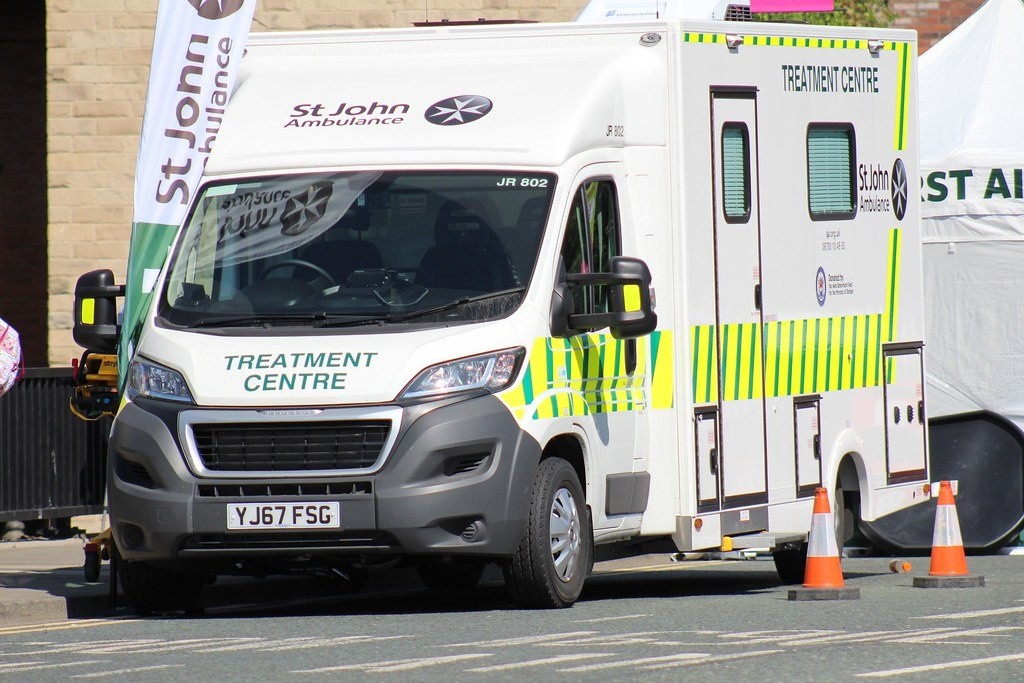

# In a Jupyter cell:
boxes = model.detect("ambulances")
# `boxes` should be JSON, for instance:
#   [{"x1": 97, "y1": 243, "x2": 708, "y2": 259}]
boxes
[{"x1": 71, "y1": 22, "x2": 932, "y2": 610}]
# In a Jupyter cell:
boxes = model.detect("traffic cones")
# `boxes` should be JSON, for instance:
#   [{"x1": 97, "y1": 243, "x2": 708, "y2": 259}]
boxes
[
  {"x1": 914, "y1": 481, "x2": 985, "y2": 589},
  {"x1": 787, "y1": 488, "x2": 861, "y2": 602}
]
